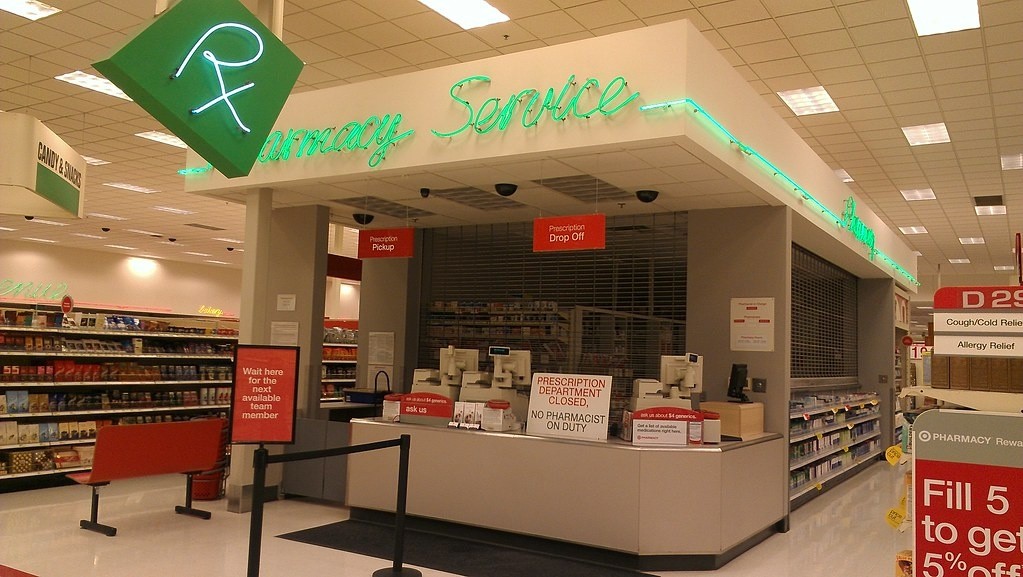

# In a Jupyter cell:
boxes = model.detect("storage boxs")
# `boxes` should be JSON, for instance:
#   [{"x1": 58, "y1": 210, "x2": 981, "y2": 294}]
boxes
[{"x1": 922, "y1": 349, "x2": 1023, "y2": 394}]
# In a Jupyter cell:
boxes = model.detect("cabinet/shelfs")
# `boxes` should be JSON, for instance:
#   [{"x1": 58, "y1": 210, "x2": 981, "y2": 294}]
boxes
[
  {"x1": 0, "y1": 325, "x2": 358, "y2": 479},
  {"x1": 790, "y1": 395, "x2": 882, "y2": 501}
]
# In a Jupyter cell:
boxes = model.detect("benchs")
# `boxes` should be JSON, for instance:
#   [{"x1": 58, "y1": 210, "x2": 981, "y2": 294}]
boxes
[{"x1": 64, "y1": 419, "x2": 227, "y2": 537}]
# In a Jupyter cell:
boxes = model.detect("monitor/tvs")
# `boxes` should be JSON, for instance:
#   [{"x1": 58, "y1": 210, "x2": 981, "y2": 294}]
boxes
[
  {"x1": 494, "y1": 350, "x2": 532, "y2": 386},
  {"x1": 661, "y1": 355, "x2": 703, "y2": 394},
  {"x1": 439, "y1": 348, "x2": 479, "y2": 384},
  {"x1": 727, "y1": 364, "x2": 747, "y2": 397}
]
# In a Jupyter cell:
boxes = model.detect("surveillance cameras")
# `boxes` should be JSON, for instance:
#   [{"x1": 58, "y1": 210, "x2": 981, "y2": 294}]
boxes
[
  {"x1": 420, "y1": 188, "x2": 430, "y2": 198},
  {"x1": 228, "y1": 248, "x2": 234, "y2": 251},
  {"x1": 102, "y1": 228, "x2": 110, "y2": 232},
  {"x1": 25, "y1": 215, "x2": 34, "y2": 220},
  {"x1": 636, "y1": 191, "x2": 658, "y2": 203},
  {"x1": 495, "y1": 184, "x2": 518, "y2": 196},
  {"x1": 353, "y1": 214, "x2": 374, "y2": 225},
  {"x1": 169, "y1": 238, "x2": 177, "y2": 242}
]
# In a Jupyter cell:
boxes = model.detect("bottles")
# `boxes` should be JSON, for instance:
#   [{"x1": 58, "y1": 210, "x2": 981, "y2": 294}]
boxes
[{"x1": 324, "y1": 365, "x2": 357, "y2": 379}]
